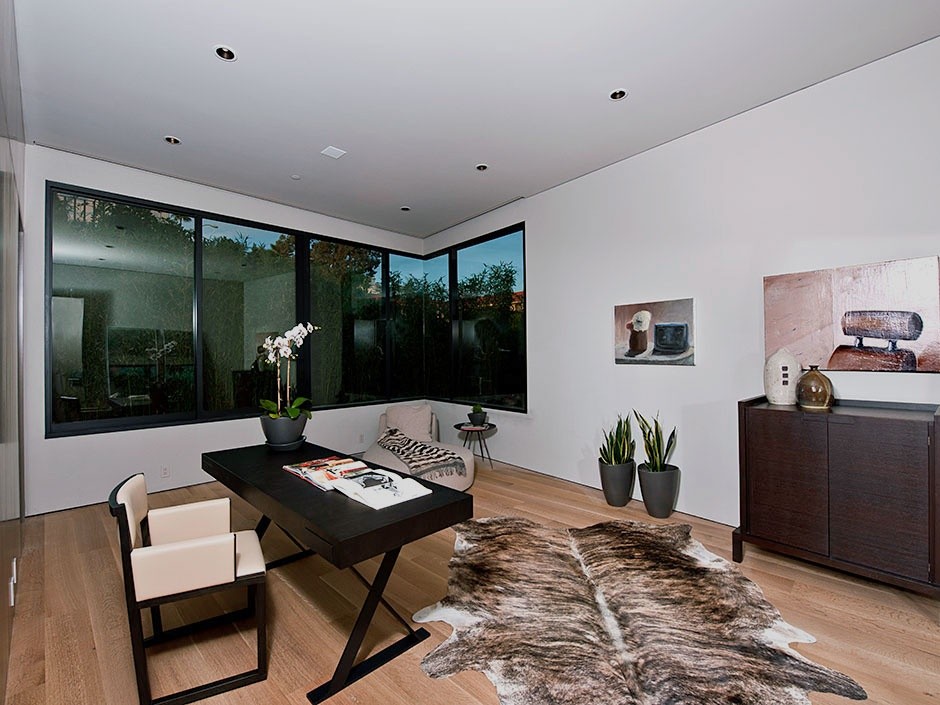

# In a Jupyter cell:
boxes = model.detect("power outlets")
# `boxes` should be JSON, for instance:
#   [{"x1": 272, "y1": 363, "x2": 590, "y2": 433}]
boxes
[{"x1": 160, "y1": 464, "x2": 170, "y2": 479}]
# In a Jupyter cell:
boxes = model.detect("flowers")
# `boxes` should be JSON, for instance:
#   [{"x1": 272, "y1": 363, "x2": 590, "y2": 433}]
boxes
[
  {"x1": 144, "y1": 341, "x2": 182, "y2": 362},
  {"x1": 258, "y1": 319, "x2": 322, "y2": 418}
]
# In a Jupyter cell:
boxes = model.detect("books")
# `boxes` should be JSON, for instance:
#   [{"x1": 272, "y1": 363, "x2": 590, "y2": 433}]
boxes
[{"x1": 281, "y1": 455, "x2": 433, "y2": 511}]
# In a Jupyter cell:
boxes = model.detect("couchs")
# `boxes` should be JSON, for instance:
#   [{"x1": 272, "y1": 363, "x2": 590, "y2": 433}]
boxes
[{"x1": 361, "y1": 412, "x2": 475, "y2": 493}]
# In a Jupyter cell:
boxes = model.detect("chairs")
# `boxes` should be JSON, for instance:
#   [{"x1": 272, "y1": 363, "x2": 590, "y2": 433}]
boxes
[
  {"x1": 52, "y1": 370, "x2": 84, "y2": 419},
  {"x1": 107, "y1": 470, "x2": 270, "y2": 705}
]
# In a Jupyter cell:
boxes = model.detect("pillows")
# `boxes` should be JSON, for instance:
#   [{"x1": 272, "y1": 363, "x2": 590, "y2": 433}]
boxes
[{"x1": 386, "y1": 400, "x2": 433, "y2": 444}]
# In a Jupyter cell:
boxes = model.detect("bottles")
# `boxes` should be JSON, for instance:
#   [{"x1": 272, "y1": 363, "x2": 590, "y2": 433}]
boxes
[{"x1": 795, "y1": 364, "x2": 833, "y2": 410}]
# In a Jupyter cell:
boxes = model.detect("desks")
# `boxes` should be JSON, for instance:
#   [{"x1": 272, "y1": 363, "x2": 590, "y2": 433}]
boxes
[
  {"x1": 200, "y1": 440, "x2": 474, "y2": 705},
  {"x1": 453, "y1": 422, "x2": 497, "y2": 470},
  {"x1": 108, "y1": 393, "x2": 155, "y2": 417}
]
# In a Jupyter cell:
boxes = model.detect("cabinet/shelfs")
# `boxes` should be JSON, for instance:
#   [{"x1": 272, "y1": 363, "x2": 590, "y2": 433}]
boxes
[
  {"x1": 732, "y1": 394, "x2": 940, "y2": 604},
  {"x1": 0, "y1": 0, "x2": 26, "y2": 705}
]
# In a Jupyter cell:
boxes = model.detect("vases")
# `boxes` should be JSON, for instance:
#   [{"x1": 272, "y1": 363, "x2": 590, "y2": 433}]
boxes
[{"x1": 259, "y1": 411, "x2": 309, "y2": 452}]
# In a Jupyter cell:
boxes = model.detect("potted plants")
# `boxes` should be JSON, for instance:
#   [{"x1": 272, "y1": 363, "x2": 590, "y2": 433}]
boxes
[
  {"x1": 597, "y1": 410, "x2": 637, "y2": 508},
  {"x1": 467, "y1": 404, "x2": 488, "y2": 428},
  {"x1": 631, "y1": 407, "x2": 681, "y2": 520}
]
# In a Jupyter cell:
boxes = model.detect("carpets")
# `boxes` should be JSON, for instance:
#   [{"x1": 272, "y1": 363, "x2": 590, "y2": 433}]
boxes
[{"x1": 410, "y1": 514, "x2": 869, "y2": 705}]
